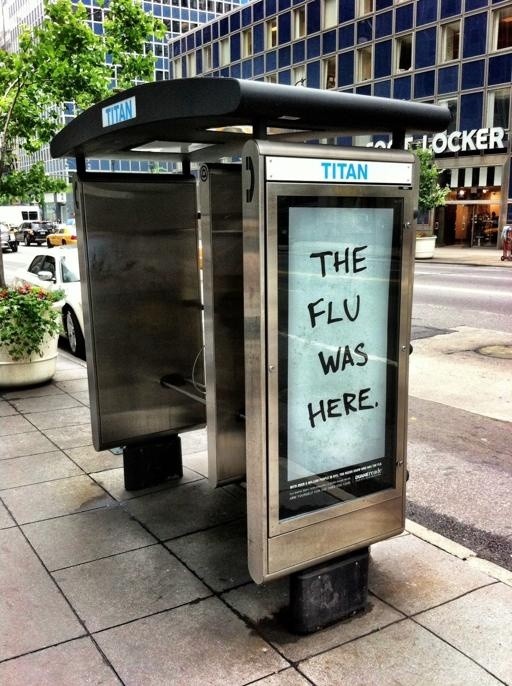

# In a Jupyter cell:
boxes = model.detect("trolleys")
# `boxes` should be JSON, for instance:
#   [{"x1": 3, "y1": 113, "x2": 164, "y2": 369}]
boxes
[{"x1": 500, "y1": 228, "x2": 512, "y2": 261}]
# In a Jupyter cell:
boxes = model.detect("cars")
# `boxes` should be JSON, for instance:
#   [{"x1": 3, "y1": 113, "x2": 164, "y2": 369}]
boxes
[{"x1": 0, "y1": 217, "x2": 86, "y2": 361}]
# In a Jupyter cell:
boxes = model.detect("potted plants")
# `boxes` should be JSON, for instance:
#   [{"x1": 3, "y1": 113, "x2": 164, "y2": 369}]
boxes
[{"x1": 408, "y1": 142, "x2": 452, "y2": 260}]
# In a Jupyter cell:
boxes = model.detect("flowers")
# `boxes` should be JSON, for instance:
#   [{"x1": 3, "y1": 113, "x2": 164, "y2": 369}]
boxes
[{"x1": 0, "y1": 280, "x2": 68, "y2": 364}]
